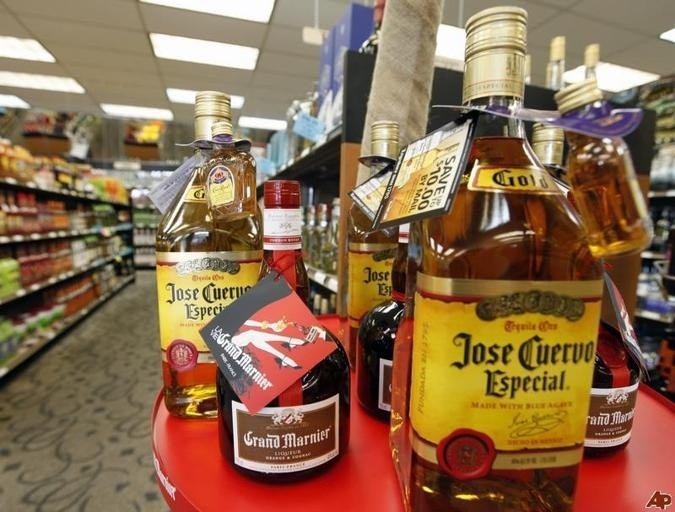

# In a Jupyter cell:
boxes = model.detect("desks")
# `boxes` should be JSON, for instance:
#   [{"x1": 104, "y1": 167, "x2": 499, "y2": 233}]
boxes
[{"x1": 149, "y1": 314, "x2": 675, "y2": 512}]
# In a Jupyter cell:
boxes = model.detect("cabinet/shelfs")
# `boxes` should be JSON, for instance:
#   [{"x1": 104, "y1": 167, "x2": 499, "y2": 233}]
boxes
[
  {"x1": 633, "y1": 75, "x2": 675, "y2": 325},
  {"x1": 0, "y1": 122, "x2": 343, "y2": 383}
]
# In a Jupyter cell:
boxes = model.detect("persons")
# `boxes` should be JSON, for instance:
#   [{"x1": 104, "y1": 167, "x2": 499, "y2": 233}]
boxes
[{"x1": 231, "y1": 318, "x2": 310, "y2": 369}]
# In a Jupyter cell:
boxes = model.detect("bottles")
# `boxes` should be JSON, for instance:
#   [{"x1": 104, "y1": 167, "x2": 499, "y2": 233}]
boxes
[
  {"x1": 357, "y1": 221, "x2": 413, "y2": 425},
  {"x1": 157, "y1": 89, "x2": 263, "y2": 419},
  {"x1": 614, "y1": 85, "x2": 675, "y2": 401},
  {"x1": 532, "y1": 123, "x2": 565, "y2": 184},
  {"x1": 215, "y1": 180, "x2": 350, "y2": 482},
  {"x1": 553, "y1": 78, "x2": 655, "y2": 264},
  {"x1": 284, "y1": 92, "x2": 342, "y2": 317},
  {"x1": 199, "y1": 120, "x2": 257, "y2": 222},
  {"x1": 586, "y1": 320, "x2": 644, "y2": 457},
  {"x1": 392, "y1": 6, "x2": 607, "y2": 512},
  {"x1": 350, "y1": 122, "x2": 399, "y2": 348}
]
